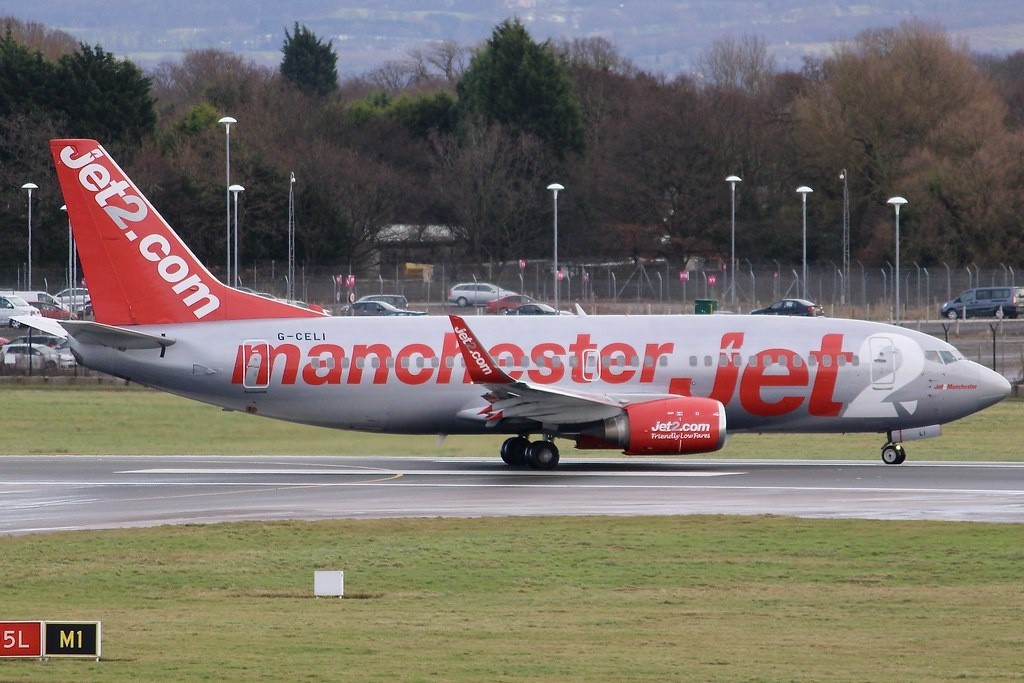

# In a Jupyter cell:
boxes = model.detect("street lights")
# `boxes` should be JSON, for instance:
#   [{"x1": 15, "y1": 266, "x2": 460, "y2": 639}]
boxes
[
  {"x1": 547, "y1": 182, "x2": 564, "y2": 316},
  {"x1": 59, "y1": 202, "x2": 72, "y2": 320},
  {"x1": 796, "y1": 185, "x2": 814, "y2": 301},
  {"x1": 21, "y1": 183, "x2": 38, "y2": 292},
  {"x1": 887, "y1": 196, "x2": 909, "y2": 328},
  {"x1": 229, "y1": 184, "x2": 245, "y2": 290},
  {"x1": 217, "y1": 116, "x2": 237, "y2": 289},
  {"x1": 725, "y1": 175, "x2": 741, "y2": 306}
]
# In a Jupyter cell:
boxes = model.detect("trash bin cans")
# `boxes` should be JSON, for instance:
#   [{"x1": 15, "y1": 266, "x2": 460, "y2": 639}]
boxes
[{"x1": 695, "y1": 299, "x2": 718, "y2": 315}]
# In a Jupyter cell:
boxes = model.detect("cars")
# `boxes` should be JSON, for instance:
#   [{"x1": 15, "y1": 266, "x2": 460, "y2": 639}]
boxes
[
  {"x1": 447, "y1": 282, "x2": 519, "y2": 307},
  {"x1": 0, "y1": 287, "x2": 95, "y2": 375},
  {"x1": 506, "y1": 303, "x2": 574, "y2": 315},
  {"x1": 339, "y1": 300, "x2": 428, "y2": 317},
  {"x1": 485, "y1": 294, "x2": 544, "y2": 316},
  {"x1": 748, "y1": 298, "x2": 825, "y2": 318}
]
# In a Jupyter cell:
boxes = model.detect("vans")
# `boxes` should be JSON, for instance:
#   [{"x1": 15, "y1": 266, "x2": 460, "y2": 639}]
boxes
[
  {"x1": 356, "y1": 294, "x2": 409, "y2": 311},
  {"x1": 940, "y1": 284, "x2": 1024, "y2": 320}
]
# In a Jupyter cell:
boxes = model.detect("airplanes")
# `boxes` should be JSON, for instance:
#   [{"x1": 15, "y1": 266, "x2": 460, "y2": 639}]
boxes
[{"x1": 11, "y1": 137, "x2": 1016, "y2": 473}]
